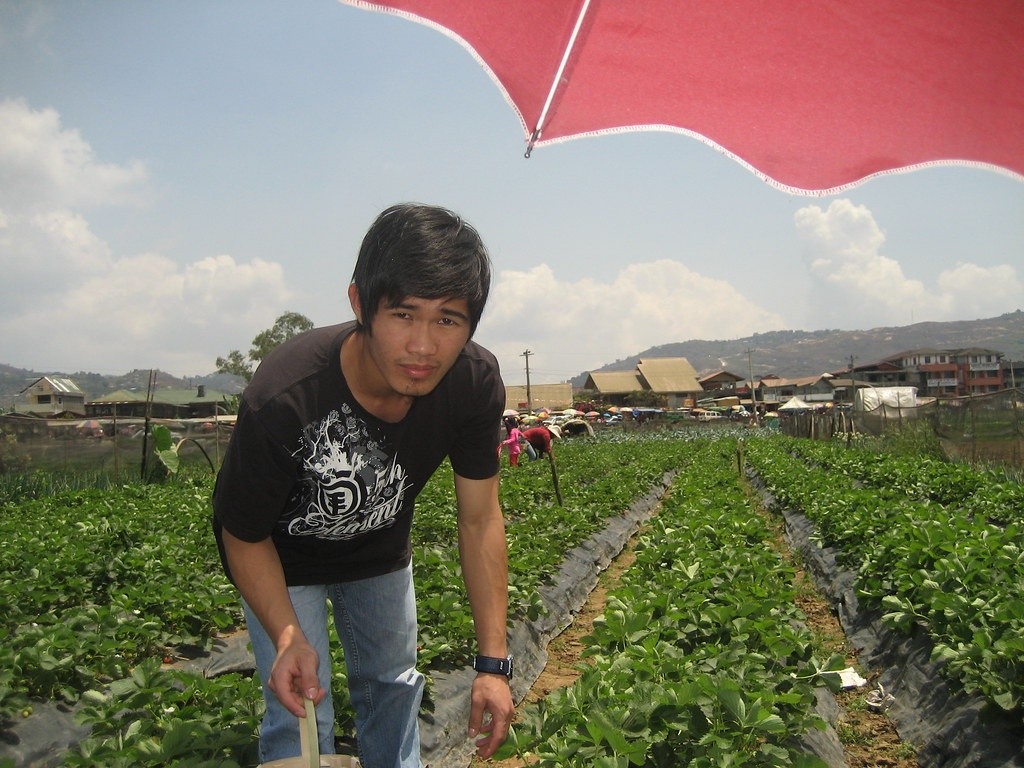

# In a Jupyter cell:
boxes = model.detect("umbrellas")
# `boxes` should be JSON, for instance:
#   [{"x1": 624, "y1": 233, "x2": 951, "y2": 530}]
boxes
[
  {"x1": 778, "y1": 396, "x2": 809, "y2": 413},
  {"x1": 344, "y1": 0, "x2": 1024, "y2": 194},
  {"x1": 764, "y1": 411, "x2": 780, "y2": 417}
]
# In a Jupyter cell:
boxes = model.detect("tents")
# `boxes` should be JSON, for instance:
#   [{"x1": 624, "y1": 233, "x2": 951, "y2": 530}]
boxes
[{"x1": 502, "y1": 408, "x2": 626, "y2": 424}]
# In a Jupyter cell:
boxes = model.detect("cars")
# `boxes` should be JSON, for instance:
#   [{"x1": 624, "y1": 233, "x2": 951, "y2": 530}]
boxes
[
  {"x1": 541, "y1": 415, "x2": 567, "y2": 426},
  {"x1": 738, "y1": 411, "x2": 760, "y2": 418}
]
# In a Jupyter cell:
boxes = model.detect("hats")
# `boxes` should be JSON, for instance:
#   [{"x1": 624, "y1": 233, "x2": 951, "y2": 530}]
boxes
[{"x1": 547, "y1": 425, "x2": 561, "y2": 440}]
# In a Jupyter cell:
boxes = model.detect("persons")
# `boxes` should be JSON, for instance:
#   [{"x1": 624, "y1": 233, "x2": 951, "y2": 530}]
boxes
[
  {"x1": 212, "y1": 204, "x2": 516, "y2": 768},
  {"x1": 522, "y1": 425, "x2": 562, "y2": 461},
  {"x1": 500, "y1": 418, "x2": 526, "y2": 468}
]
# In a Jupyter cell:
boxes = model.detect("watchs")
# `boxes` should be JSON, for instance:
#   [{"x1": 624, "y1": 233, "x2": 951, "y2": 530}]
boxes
[{"x1": 472, "y1": 654, "x2": 515, "y2": 681}]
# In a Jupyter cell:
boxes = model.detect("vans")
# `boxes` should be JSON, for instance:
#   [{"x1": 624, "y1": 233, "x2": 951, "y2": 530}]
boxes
[{"x1": 698, "y1": 411, "x2": 723, "y2": 423}]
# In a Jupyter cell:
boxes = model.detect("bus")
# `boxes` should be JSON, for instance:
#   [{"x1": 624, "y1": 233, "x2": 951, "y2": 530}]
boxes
[{"x1": 831, "y1": 386, "x2": 856, "y2": 412}]
